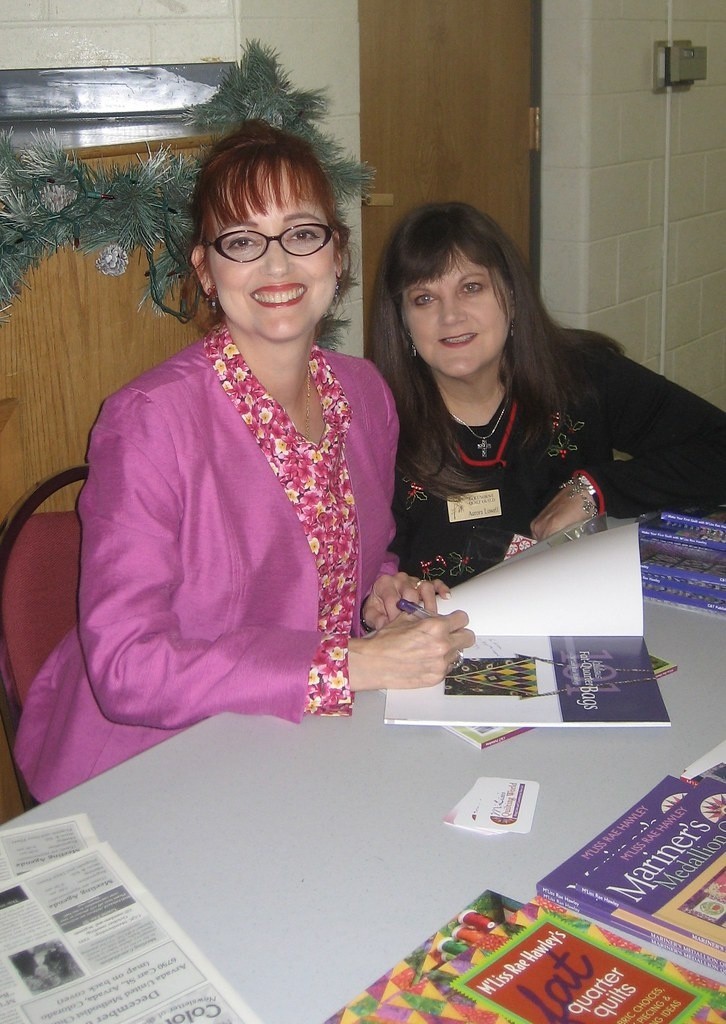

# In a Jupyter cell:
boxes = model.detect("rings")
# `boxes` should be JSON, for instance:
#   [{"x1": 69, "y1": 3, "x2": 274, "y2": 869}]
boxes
[
  {"x1": 414, "y1": 580, "x2": 426, "y2": 589},
  {"x1": 453, "y1": 650, "x2": 464, "y2": 666}
]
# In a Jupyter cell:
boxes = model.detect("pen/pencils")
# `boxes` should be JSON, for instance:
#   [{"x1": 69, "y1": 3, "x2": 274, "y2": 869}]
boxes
[{"x1": 397, "y1": 599, "x2": 438, "y2": 621}]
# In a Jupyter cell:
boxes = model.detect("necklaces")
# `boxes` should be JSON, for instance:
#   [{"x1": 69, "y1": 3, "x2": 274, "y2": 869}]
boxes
[
  {"x1": 303, "y1": 366, "x2": 312, "y2": 440},
  {"x1": 449, "y1": 405, "x2": 506, "y2": 458}
]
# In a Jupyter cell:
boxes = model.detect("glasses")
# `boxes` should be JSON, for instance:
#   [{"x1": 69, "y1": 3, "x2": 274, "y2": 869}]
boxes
[{"x1": 201, "y1": 223, "x2": 334, "y2": 263}]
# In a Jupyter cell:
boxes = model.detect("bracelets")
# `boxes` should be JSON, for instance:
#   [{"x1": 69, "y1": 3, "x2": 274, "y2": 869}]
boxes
[{"x1": 560, "y1": 473, "x2": 600, "y2": 519}]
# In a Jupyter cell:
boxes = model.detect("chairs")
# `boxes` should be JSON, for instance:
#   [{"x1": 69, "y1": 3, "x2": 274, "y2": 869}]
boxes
[{"x1": 0, "y1": 464, "x2": 90, "y2": 813}]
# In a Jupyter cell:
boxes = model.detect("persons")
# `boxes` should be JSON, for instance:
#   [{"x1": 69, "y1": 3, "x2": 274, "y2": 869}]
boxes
[
  {"x1": 10, "y1": 117, "x2": 475, "y2": 803},
  {"x1": 368, "y1": 200, "x2": 726, "y2": 593}
]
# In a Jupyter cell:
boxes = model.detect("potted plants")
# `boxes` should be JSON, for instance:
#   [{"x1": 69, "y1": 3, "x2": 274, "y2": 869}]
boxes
[{"x1": 0, "y1": 38, "x2": 374, "y2": 356}]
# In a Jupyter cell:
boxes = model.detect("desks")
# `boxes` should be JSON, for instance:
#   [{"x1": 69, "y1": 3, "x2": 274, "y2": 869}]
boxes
[{"x1": 0, "y1": 602, "x2": 726, "y2": 1024}]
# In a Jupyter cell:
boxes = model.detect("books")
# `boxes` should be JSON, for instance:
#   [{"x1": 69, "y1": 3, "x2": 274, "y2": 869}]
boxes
[
  {"x1": 636, "y1": 497, "x2": 726, "y2": 616},
  {"x1": 381, "y1": 520, "x2": 678, "y2": 750},
  {"x1": 325, "y1": 739, "x2": 726, "y2": 1024}
]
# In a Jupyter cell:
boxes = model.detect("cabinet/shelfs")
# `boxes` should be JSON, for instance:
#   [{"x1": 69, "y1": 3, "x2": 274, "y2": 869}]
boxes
[{"x1": 0, "y1": 62, "x2": 266, "y2": 523}]
